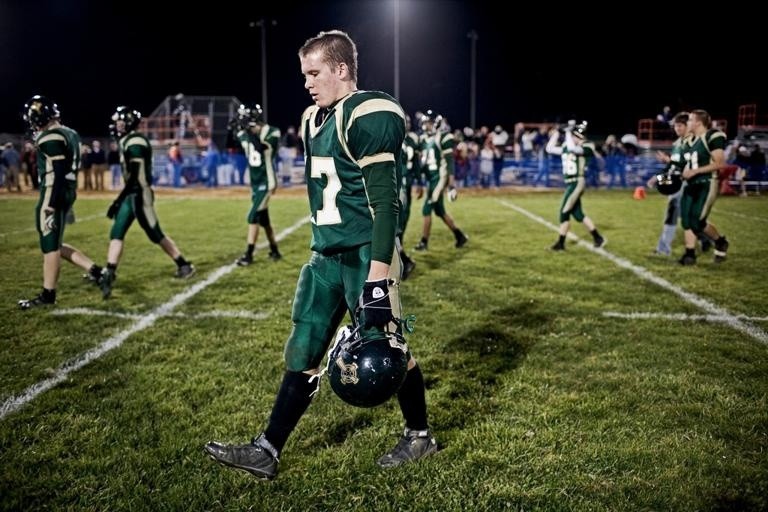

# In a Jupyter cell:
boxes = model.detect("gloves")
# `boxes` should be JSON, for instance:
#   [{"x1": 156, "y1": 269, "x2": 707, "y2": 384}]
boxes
[
  {"x1": 353, "y1": 278, "x2": 396, "y2": 332},
  {"x1": 107, "y1": 200, "x2": 120, "y2": 219},
  {"x1": 447, "y1": 184, "x2": 457, "y2": 201}
]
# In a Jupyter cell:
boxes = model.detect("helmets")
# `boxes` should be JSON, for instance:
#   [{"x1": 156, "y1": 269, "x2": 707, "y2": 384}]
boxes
[
  {"x1": 326, "y1": 314, "x2": 417, "y2": 408},
  {"x1": 655, "y1": 174, "x2": 683, "y2": 195},
  {"x1": 236, "y1": 102, "x2": 262, "y2": 130},
  {"x1": 561, "y1": 119, "x2": 588, "y2": 139},
  {"x1": 22, "y1": 94, "x2": 61, "y2": 132},
  {"x1": 108, "y1": 105, "x2": 142, "y2": 141},
  {"x1": 417, "y1": 109, "x2": 442, "y2": 135}
]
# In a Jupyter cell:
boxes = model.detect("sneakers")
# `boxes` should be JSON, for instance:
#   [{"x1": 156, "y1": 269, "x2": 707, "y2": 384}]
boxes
[
  {"x1": 99, "y1": 267, "x2": 115, "y2": 299},
  {"x1": 236, "y1": 256, "x2": 253, "y2": 266},
  {"x1": 204, "y1": 430, "x2": 280, "y2": 480},
  {"x1": 702, "y1": 241, "x2": 711, "y2": 251},
  {"x1": 714, "y1": 240, "x2": 728, "y2": 261},
  {"x1": 82, "y1": 271, "x2": 117, "y2": 282},
  {"x1": 678, "y1": 256, "x2": 696, "y2": 265},
  {"x1": 16, "y1": 294, "x2": 56, "y2": 310},
  {"x1": 456, "y1": 236, "x2": 467, "y2": 247},
  {"x1": 172, "y1": 263, "x2": 195, "y2": 280},
  {"x1": 269, "y1": 252, "x2": 281, "y2": 260},
  {"x1": 593, "y1": 236, "x2": 605, "y2": 248},
  {"x1": 551, "y1": 245, "x2": 564, "y2": 250},
  {"x1": 376, "y1": 429, "x2": 438, "y2": 466},
  {"x1": 414, "y1": 242, "x2": 425, "y2": 249},
  {"x1": 402, "y1": 260, "x2": 416, "y2": 281}
]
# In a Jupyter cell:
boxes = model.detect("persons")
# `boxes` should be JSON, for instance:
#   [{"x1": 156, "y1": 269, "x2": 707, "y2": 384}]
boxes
[
  {"x1": 83, "y1": 106, "x2": 196, "y2": 281},
  {"x1": 17, "y1": 95, "x2": 106, "y2": 307},
  {"x1": 393, "y1": 114, "x2": 467, "y2": 276},
  {"x1": 203, "y1": 30, "x2": 437, "y2": 480},
  {"x1": 647, "y1": 110, "x2": 729, "y2": 268},
  {"x1": 227, "y1": 102, "x2": 281, "y2": 266},
  {"x1": 1, "y1": 94, "x2": 768, "y2": 201},
  {"x1": 546, "y1": 119, "x2": 606, "y2": 251}
]
